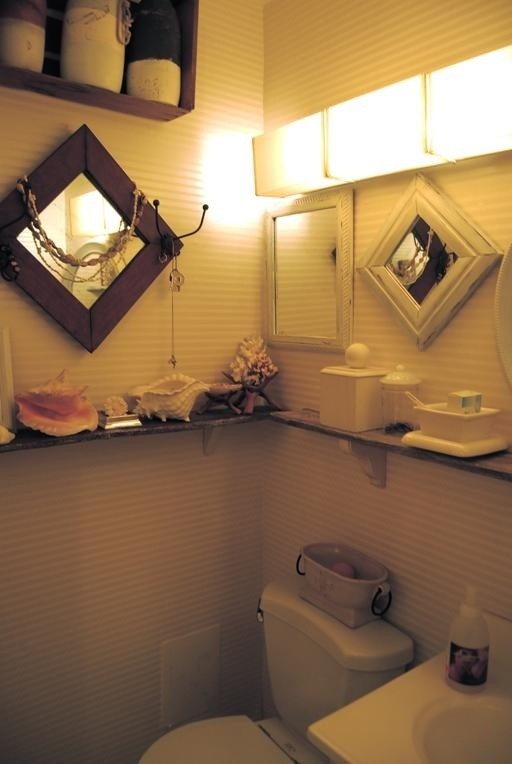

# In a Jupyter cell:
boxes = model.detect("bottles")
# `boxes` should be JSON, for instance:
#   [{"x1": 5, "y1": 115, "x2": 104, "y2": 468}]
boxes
[
  {"x1": 1, "y1": 0, "x2": 47, "y2": 72},
  {"x1": 379, "y1": 364, "x2": 422, "y2": 435},
  {"x1": 442, "y1": 600, "x2": 489, "y2": 693},
  {"x1": 60, "y1": 0, "x2": 126, "y2": 93}
]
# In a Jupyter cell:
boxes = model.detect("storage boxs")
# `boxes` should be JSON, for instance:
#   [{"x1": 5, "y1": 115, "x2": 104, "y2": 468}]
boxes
[{"x1": 317, "y1": 342, "x2": 389, "y2": 434}]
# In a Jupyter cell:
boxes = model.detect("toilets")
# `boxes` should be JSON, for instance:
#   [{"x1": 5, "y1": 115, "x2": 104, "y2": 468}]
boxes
[{"x1": 137, "y1": 575, "x2": 414, "y2": 764}]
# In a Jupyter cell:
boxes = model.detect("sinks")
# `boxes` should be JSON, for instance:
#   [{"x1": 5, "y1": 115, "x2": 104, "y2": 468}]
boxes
[{"x1": 410, "y1": 687, "x2": 512, "y2": 763}]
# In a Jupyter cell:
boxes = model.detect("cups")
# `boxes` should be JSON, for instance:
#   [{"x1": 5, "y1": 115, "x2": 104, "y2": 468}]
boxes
[{"x1": 128, "y1": 59, "x2": 181, "y2": 104}]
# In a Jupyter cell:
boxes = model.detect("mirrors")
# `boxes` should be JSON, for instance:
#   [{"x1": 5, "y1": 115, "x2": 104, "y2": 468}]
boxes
[
  {"x1": 260, "y1": 185, "x2": 356, "y2": 359},
  {"x1": 0, "y1": 119, "x2": 186, "y2": 357},
  {"x1": 353, "y1": 170, "x2": 504, "y2": 351},
  {"x1": 387, "y1": 218, "x2": 450, "y2": 304}
]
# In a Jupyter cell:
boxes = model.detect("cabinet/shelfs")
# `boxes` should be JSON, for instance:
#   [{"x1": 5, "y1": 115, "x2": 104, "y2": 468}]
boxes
[{"x1": 0, "y1": 0, "x2": 201, "y2": 123}]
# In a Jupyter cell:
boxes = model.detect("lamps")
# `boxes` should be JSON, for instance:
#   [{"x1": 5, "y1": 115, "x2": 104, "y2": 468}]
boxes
[
  {"x1": 325, "y1": 73, "x2": 451, "y2": 183},
  {"x1": 68, "y1": 189, "x2": 132, "y2": 237},
  {"x1": 425, "y1": 43, "x2": 512, "y2": 162},
  {"x1": 252, "y1": 110, "x2": 348, "y2": 198}
]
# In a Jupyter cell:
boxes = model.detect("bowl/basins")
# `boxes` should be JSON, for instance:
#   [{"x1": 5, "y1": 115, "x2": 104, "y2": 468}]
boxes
[{"x1": 414, "y1": 401, "x2": 501, "y2": 441}]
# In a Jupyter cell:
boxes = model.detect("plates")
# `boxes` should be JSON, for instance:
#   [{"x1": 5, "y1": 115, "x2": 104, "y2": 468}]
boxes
[{"x1": 401, "y1": 430, "x2": 510, "y2": 457}]
